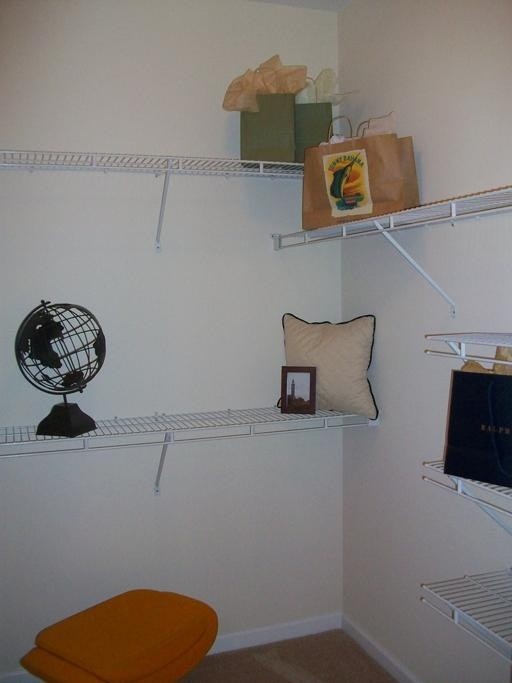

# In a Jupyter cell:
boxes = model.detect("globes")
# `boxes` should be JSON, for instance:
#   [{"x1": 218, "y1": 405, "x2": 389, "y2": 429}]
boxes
[{"x1": 15, "y1": 300, "x2": 106, "y2": 438}]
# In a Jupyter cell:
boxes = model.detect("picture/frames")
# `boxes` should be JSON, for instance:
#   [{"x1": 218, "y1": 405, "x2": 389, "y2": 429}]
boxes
[{"x1": 281, "y1": 367, "x2": 316, "y2": 414}]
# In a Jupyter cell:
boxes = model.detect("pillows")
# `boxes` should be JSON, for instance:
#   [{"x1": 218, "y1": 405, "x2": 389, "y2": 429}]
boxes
[{"x1": 277, "y1": 313, "x2": 378, "y2": 420}]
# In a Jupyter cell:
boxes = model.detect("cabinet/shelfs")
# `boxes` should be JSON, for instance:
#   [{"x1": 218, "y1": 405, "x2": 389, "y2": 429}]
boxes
[
  {"x1": 0, "y1": 150, "x2": 382, "y2": 492},
  {"x1": 272, "y1": 185, "x2": 512, "y2": 663}
]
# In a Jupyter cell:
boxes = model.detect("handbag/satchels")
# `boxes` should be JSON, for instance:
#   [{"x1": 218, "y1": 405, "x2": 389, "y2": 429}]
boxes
[{"x1": 301, "y1": 115, "x2": 419, "y2": 229}]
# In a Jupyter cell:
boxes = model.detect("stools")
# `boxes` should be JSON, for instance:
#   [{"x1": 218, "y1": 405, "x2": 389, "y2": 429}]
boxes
[{"x1": 20, "y1": 590, "x2": 218, "y2": 683}]
